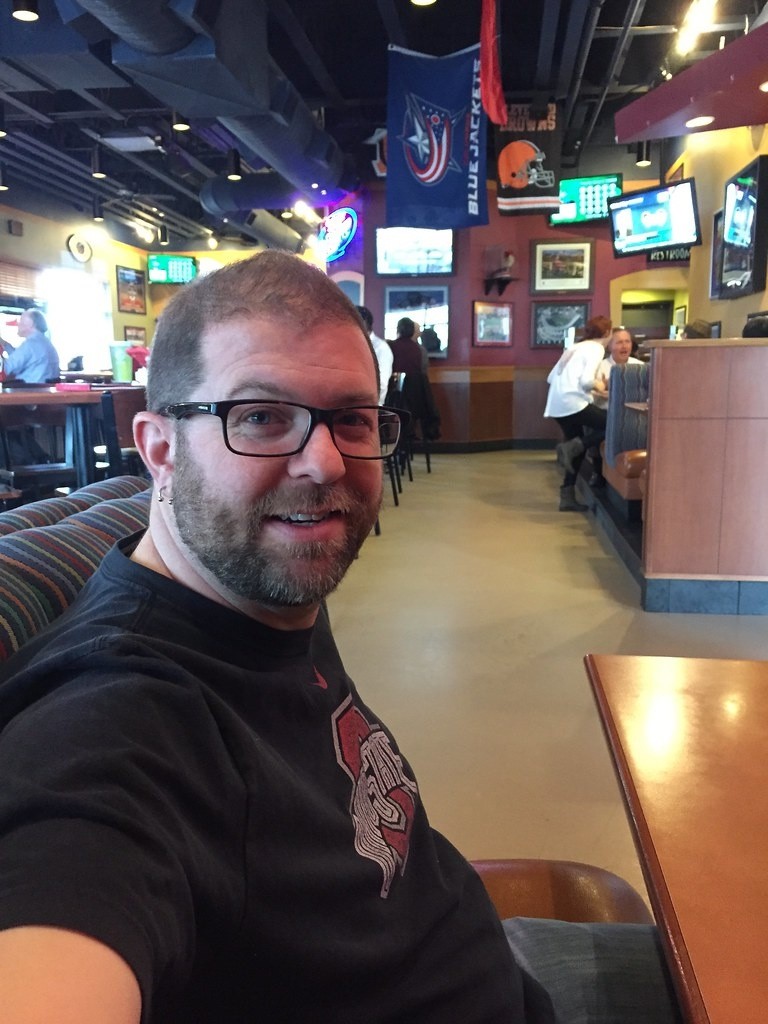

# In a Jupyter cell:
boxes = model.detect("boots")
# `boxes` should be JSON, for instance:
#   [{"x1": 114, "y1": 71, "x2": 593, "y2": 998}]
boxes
[
  {"x1": 556, "y1": 438, "x2": 585, "y2": 473},
  {"x1": 558, "y1": 483, "x2": 589, "y2": 512}
]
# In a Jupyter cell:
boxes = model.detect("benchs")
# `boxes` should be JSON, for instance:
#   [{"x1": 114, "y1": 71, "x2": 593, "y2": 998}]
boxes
[
  {"x1": 0, "y1": 475, "x2": 656, "y2": 932},
  {"x1": 600, "y1": 364, "x2": 648, "y2": 520}
]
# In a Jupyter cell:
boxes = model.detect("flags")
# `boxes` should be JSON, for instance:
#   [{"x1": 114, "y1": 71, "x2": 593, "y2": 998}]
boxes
[{"x1": 383, "y1": 35, "x2": 500, "y2": 230}]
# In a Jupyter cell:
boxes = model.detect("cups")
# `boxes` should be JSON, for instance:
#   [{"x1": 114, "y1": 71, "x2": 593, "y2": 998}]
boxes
[{"x1": 108, "y1": 341, "x2": 135, "y2": 384}]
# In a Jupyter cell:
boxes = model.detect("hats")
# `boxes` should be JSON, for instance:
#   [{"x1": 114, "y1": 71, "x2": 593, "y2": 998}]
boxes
[{"x1": 685, "y1": 319, "x2": 713, "y2": 338}]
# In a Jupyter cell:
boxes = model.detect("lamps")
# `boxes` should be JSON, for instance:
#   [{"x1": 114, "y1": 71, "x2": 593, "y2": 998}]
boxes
[
  {"x1": 281, "y1": 208, "x2": 292, "y2": 218},
  {"x1": 11, "y1": 0, "x2": 40, "y2": 22},
  {"x1": 93, "y1": 110, "x2": 242, "y2": 247},
  {"x1": 637, "y1": 141, "x2": 651, "y2": 167},
  {"x1": 0, "y1": 103, "x2": 9, "y2": 191}
]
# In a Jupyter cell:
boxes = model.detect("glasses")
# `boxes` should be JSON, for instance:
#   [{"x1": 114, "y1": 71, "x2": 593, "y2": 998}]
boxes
[{"x1": 156, "y1": 398, "x2": 411, "y2": 461}]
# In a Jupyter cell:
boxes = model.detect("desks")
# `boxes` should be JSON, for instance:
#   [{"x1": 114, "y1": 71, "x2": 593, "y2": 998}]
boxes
[
  {"x1": 583, "y1": 652, "x2": 768, "y2": 1024},
  {"x1": 624, "y1": 403, "x2": 648, "y2": 416},
  {"x1": 591, "y1": 391, "x2": 609, "y2": 399},
  {"x1": 0, "y1": 387, "x2": 106, "y2": 489}
]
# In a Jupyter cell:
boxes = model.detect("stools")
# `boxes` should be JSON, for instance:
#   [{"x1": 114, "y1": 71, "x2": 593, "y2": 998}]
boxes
[{"x1": 375, "y1": 372, "x2": 431, "y2": 536}]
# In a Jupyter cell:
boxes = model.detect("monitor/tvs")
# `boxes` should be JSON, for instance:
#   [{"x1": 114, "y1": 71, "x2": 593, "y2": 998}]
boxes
[
  {"x1": 148, "y1": 253, "x2": 198, "y2": 284},
  {"x1": 374, "y1": 227, "x2": 456, "y2": 278},
  {"x1": 608, "y1": 177, "x2": 702, "y2": 258},
  {"x1": 549, "y1": 173, "x2": 623, "y2": 226},
  {"x1": 722, "y1": 179, "x2": 756, "y2": 250}
]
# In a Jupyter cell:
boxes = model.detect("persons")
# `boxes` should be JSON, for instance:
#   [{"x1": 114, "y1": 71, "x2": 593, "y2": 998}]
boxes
[
  {"x1": 355, "y1": 305, "x2": 395, "y2": 406},
  {"x1": 0, "y1": 251, "x2": 555, "y2": 1024},
  {"x1": 742, "y1": 315, "x2": 768, "y2": 337},
  {"x1": 681, "y1": 319, "x2": 712, "y2": 339},
  {"x1": 0, "y1": 308, "x2": 60, "y2": 465},
  {"x1": 543, "y1": 314, "x2": 642, "y2": 513},
  {"x1": 389, "y1": 318, "x2": 429, "y2": 442}
]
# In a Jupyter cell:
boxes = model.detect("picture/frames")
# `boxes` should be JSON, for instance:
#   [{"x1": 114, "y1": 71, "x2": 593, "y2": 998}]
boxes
[
  {"x1": 472, "y1": 301, "x2": 515, "y2": 348},
  {"x1": 531, "y1": 299, "x2": 592, "y2": 349},
  {"x1": 529, "y1": 237, "x2": 596, "y2": 295},
  {"x1": 708, "y1": 207, "x2": 723, "y2": 300},
  {"x1": 116, "y1": 265, "x2": 146, "y2": 314}
]
